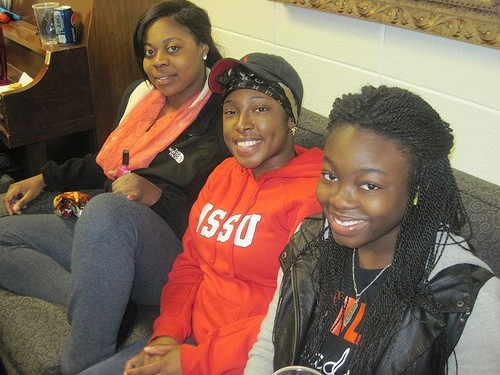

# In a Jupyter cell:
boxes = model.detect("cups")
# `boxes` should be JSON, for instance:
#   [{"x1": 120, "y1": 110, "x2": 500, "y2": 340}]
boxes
[{"x1": 32, "y1": 2, "x2": 61, "y2": 47}]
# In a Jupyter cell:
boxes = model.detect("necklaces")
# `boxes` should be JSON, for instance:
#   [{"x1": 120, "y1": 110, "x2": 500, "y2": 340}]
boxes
[{"x1": 343, "y1": 246, "x2": 393, "y2": 327}]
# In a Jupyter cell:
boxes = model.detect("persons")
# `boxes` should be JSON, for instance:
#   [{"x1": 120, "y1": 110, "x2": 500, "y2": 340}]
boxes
[
  {"x1": 69, "y1": 53, "x2": 326, "y2": 374},
  {"x1": 244, "y1": 85, "x2": 500, "y2": 375},
  {"x1": 0, "y1": 0, "x2": 235, "y2": 375}
]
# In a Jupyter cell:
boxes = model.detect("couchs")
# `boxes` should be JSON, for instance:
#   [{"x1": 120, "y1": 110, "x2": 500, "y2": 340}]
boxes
[{"x1": 0, "y1": 77, "x2": 500, "y2": 375}]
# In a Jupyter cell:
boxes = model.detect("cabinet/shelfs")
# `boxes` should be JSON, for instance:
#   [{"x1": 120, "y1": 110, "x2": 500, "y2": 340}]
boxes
[{"x1": 0, "y1": 0, "x2": 161, "y2": 177}]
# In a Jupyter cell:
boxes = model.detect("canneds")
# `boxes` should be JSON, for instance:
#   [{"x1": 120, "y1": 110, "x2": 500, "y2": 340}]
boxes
[{"x1": 53, "y1": 6, "x2": 77, "y2": 47}]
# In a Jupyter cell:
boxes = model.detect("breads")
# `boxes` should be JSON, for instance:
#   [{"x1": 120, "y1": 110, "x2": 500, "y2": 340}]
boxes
[{"x1": 53, "y1": 191, "x2": 95, "y2": 219}]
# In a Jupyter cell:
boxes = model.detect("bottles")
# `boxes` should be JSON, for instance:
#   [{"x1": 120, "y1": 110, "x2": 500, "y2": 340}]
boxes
[{"x1": 118, "y1": 150, "x2": 133, "y2": 178}]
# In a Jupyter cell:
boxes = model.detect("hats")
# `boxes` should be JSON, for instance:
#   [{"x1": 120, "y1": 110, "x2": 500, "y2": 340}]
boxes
[{"x1": 206, "y1": 53, "x2": 304, "y2": 112}]
